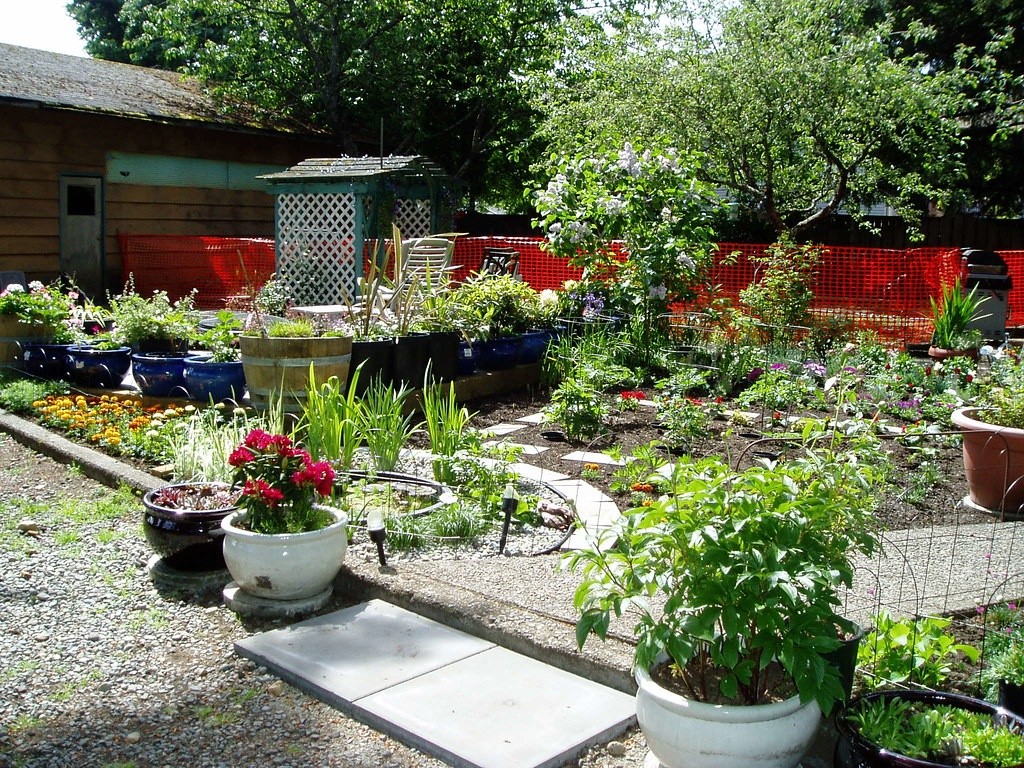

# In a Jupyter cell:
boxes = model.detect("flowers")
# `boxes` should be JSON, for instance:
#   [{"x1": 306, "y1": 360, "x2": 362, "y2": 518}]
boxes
[
  {"x1": 228, "y1": 428, "x2": 334, "y2": 533},
  {"x1": 0, "y1": 280, "x2": 80, "y2": 315}
]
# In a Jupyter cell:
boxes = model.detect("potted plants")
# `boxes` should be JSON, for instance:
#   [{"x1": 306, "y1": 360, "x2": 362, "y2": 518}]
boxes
[
  {"x1": 40, "y1": 321, "x2": 80, "y2": 383},
  {"x1": 553, "y1": 338, "x2": 893, "y2": 768},
  {"x1": 16, "y1": 304, "x2": 54, "y2": 378},
  {"x1": 950, "y1": 352, "x2": 1024, "y2": 512},
  {"x1": 182, "y1": 307, "x2": 248, "y2": 405},
  {"x1": 132, "y1": 309, "x2": 199, "y2": 396},
  {"x1": 342, "y1": 223, "x2": 550, "y2": 402},
  {"x1": 142, "y1": 481, "x2": 244, "y2": 571},
  {"x1": 915, "y1": 274, "x2": 994, "y2": 366},
  {"x1": 240, "y1": 312, "x2": 353, "y2": 425},
  {"x1": 987, "y1": 638, "x2": 1024, "y2": 721},
  {"x1": 832, "y1": 691, "x2": 1024, "y2": 768},
  {"x1": 64, "y1": 306, "x2": 131, "y2": 389}
]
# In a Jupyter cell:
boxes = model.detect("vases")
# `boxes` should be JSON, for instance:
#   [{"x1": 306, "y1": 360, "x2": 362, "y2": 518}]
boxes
[
  {"x1": 782, "y1": 613, "x2": 865, "y2": 720},
  {"x1": 0, "y1": 316, "x2": 50, "y2": 376},
  {"x1": 220, "y1": 503, "x2": 348, "y2": 601}
]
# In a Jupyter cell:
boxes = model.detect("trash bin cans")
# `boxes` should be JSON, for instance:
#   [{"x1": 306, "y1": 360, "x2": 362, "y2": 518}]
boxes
[{"x1": 959, "y1": 249, "x2": 1013, "y2": 341}]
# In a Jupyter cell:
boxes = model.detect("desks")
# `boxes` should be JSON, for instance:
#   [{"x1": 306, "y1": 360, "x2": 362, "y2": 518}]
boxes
[{"x1": 291, "y1": 305, "x2": 362, "y2": 328}]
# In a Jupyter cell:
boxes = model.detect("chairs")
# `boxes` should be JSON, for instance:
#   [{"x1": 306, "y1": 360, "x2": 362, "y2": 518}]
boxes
[
  {"x1": 476, "y1": 244, "x2": 521, "y2": 282},
  {"x1": 357, "y1": 237, "x2": 455, "y2": 314}
]
